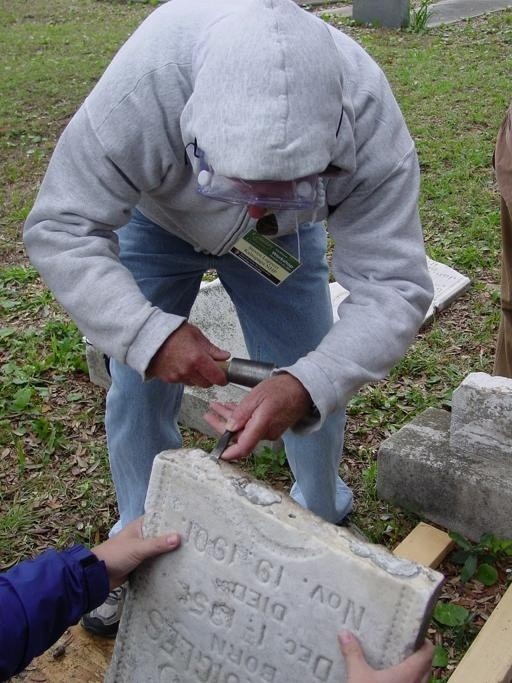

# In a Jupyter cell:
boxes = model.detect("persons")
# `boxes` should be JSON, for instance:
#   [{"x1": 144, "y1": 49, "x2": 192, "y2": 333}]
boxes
[
  {"x1": 0, "y1": 518, "x2": 435, "y2": 683},
  {"x1": 23, "y1": 0, "x2": 434, "y2": 637}
]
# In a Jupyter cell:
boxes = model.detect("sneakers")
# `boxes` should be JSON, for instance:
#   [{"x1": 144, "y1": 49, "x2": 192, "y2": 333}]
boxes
[{"x1": 81, "y1": 579, "x2": 127, "y2": 634}]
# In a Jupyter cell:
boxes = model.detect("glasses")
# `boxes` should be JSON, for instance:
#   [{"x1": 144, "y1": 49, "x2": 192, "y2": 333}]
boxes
[{"x1": 194, "y1": 154, "x2": 321, "y2": 211}]
